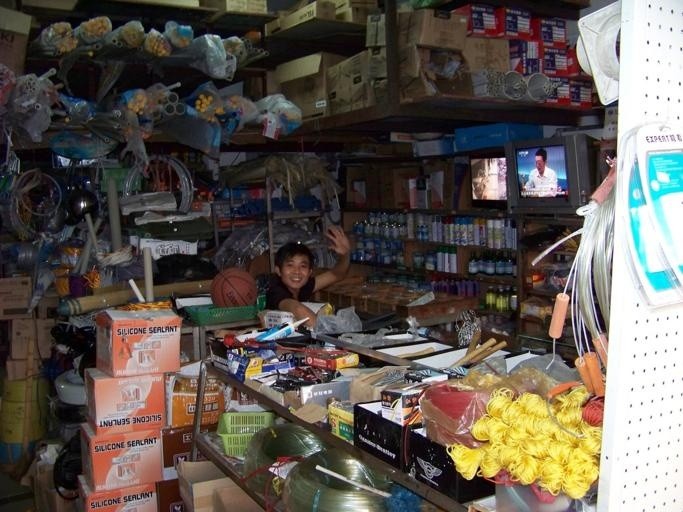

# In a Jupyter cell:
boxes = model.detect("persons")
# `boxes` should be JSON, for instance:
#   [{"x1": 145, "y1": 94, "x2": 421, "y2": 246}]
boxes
[
  {"x1": 521, "y1": 148, "x2": 558, "y2": 197},
  {"x1": 264, "y1": 224, "x2": 352, "y2": 327}
]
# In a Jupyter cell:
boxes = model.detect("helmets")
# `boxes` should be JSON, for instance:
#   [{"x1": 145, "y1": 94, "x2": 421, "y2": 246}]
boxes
[{"x1": 62, "y1": 188, "x2": 99, "y2": 225}]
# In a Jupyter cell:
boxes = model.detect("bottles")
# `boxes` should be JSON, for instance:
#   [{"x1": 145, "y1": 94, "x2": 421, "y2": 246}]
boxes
[
  {"x1": 350, "y1": 209, "x2": 519, "y2": 314},
  {"x1": 257, "y1": 317, "x2": 308, "y2": 344}
]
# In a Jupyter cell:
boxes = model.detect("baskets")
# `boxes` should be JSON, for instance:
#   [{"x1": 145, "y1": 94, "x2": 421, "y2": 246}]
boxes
[
  {"x1": 216, "y1": 412, "x2": 279, "y2": 457},
  {"x1": 184, "y1": 304, "x2": 263, "y2": 326}
]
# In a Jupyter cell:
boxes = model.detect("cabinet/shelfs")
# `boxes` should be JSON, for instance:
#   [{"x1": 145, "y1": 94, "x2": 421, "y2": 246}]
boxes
[
  {"x1": 0, "y1": 0, "x2": 278, "y2": 159},
  {"x1": 210, "y1": 170, "x2": 327, "y2": 279},
  {"x1": 189, "y1": 358, "x2": 475, "y2": 512},
  {"x1": 333, "y1": 160, "x2": 598, "y2": 338}
]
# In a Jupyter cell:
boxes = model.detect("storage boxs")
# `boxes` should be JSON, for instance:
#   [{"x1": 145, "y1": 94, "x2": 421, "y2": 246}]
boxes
[
  {"x1": 0, "y1": 6, "x2": 41, "y2": 100},
  {"x1": 218, "y1": 0, "x2": 598, "y2": 156},
  {"x1": 329, "y1": 367, "x2": 496, "y2": 504},
  {"x1": 123, "y1": 226, "x2": 201, "y2": 262},
  {"x1": 1, "y1": 270, "x2": 265, "y2": 511}
]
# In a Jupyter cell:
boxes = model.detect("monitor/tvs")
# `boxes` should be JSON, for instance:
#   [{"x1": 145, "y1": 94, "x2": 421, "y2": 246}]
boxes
[
  {"x1": 469, "y1": 146, "x2": 508, "y2": 208},
  {"x1": 505, "y1": 133, "x2": 600, "y2": 216}
]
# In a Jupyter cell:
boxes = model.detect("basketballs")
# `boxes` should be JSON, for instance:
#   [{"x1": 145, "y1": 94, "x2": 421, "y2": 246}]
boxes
[{"x1": 209, "y1": 268, "x2": 257, "y2": 306}]
